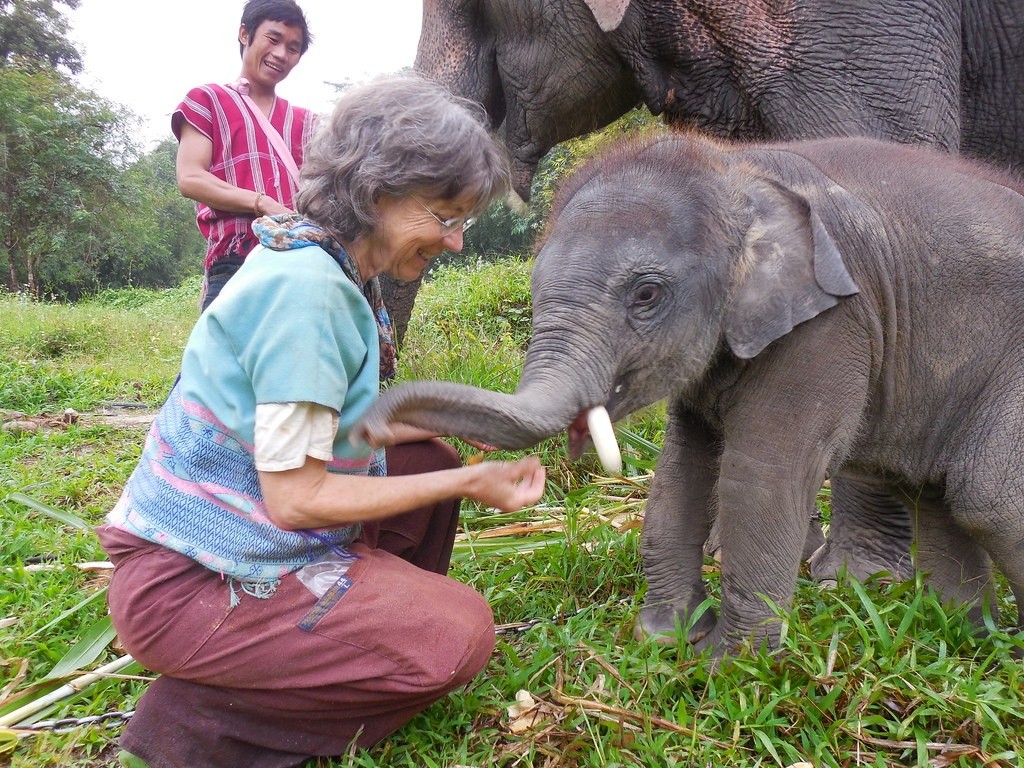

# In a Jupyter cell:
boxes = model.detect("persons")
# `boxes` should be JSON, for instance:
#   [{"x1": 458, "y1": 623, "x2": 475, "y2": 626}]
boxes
[
  {"x1": 96, "y1": 74, "x2": 547, "y2": 768},
  {"x1": 171, "y1": 0, "x2": 323, "y2": 315}
]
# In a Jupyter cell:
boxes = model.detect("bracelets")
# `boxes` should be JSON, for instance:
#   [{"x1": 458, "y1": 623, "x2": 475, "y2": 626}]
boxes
[{"x1": 254, "y1": 193, "x2": 265, "y2": 217}]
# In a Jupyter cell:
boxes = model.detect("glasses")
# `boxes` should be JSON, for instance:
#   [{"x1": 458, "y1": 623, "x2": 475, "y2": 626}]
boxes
[{"x1": 405, "y1": 190, "x2": 478, "y2": 237}]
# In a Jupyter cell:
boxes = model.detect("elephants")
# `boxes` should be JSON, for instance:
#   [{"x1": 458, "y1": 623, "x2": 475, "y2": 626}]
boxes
[
  {"x1": 348, "y1": 138, "x2": 1021, "y2": 658},
  {"x1": 415, "y1": 1, "x2": 1023, "y2": 585}
]
